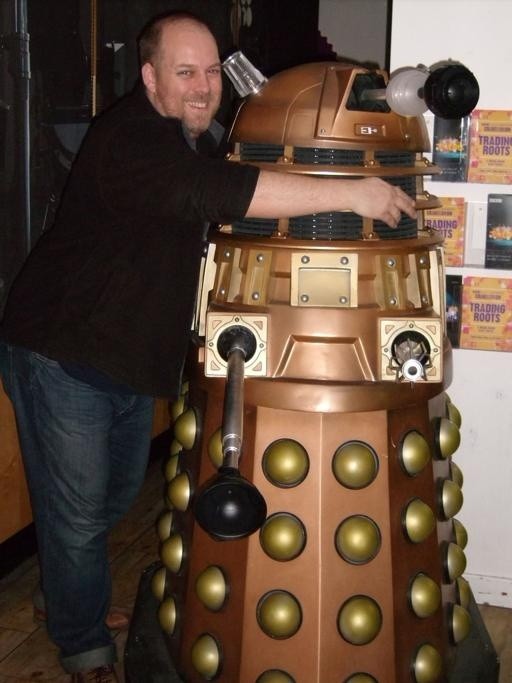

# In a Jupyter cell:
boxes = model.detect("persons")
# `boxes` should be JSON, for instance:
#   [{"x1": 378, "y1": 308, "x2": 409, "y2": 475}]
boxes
[{"x1": 0, "y1": 12, "x2": 416, "y2": 681}]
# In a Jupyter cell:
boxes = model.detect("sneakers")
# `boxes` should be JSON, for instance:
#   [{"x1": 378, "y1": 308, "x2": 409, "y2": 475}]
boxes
[
  {"x1": 32, "y1": 604, "x2": 133, "y2": 629},
  {"x1": 71, "y1": 663, "x2": 119, "y2": 683}
]
